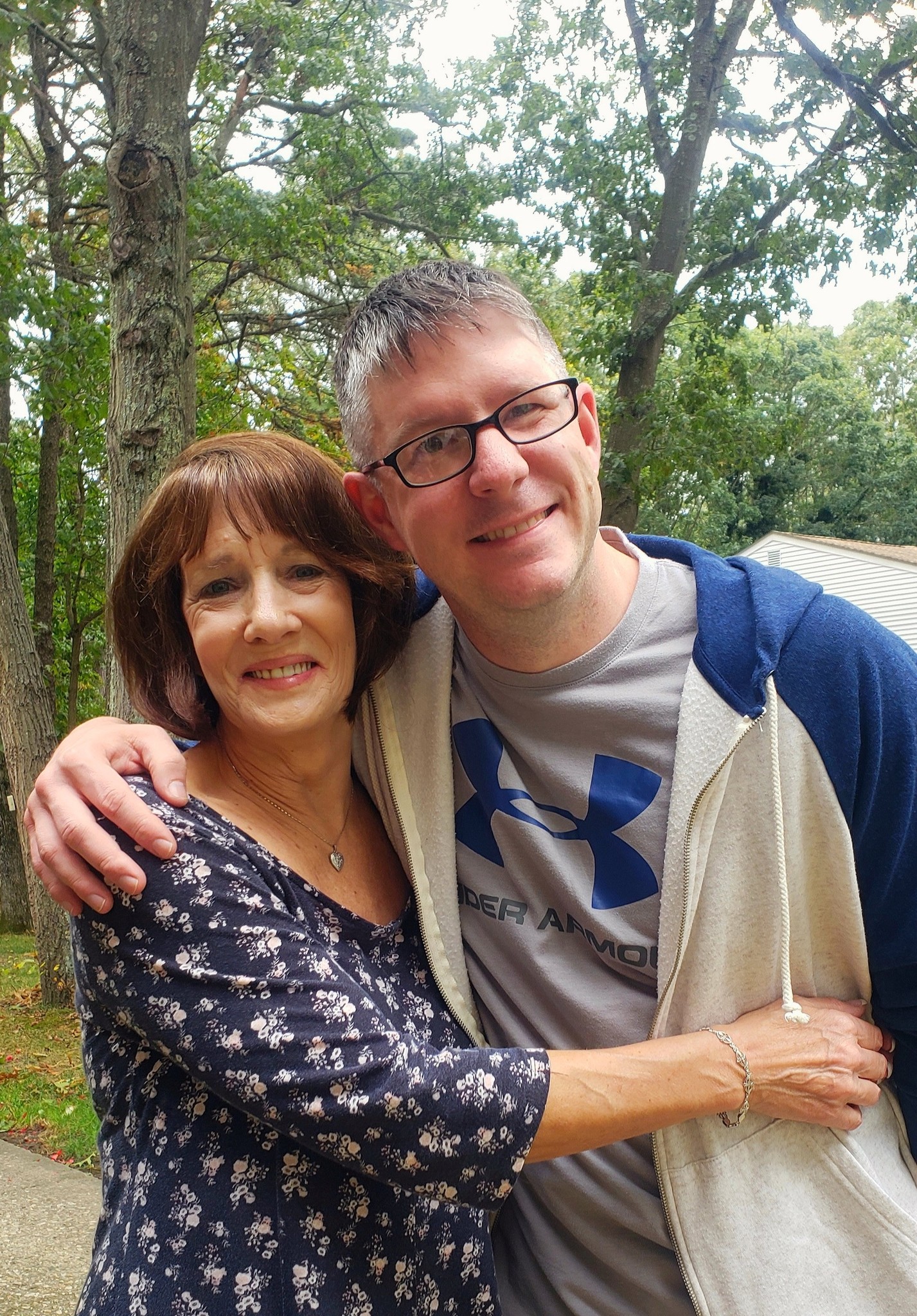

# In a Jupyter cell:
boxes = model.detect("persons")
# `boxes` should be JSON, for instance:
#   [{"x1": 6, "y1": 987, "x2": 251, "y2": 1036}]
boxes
[
  {"x1": 14, "y1": 255, "x2": 917, "y2": 1310},
  {"x1": 27, "y1": 426, "x2": 899, "y2": 1315}
]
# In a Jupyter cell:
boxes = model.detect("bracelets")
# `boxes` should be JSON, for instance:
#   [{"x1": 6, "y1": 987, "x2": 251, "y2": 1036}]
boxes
[{"x1": 698, "y1": 1026, "x2": 753, "y2": 1127}]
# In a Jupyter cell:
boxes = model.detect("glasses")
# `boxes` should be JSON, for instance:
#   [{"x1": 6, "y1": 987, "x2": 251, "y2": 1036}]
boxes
[{"x1": 356, "y1": 378, "x2": 578, "y2": 488}]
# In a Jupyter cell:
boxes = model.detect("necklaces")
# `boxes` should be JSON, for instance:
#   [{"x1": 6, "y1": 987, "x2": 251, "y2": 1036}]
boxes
[{"x1": 216, "y1": 734, "x2": 356, "y2": 873}]
